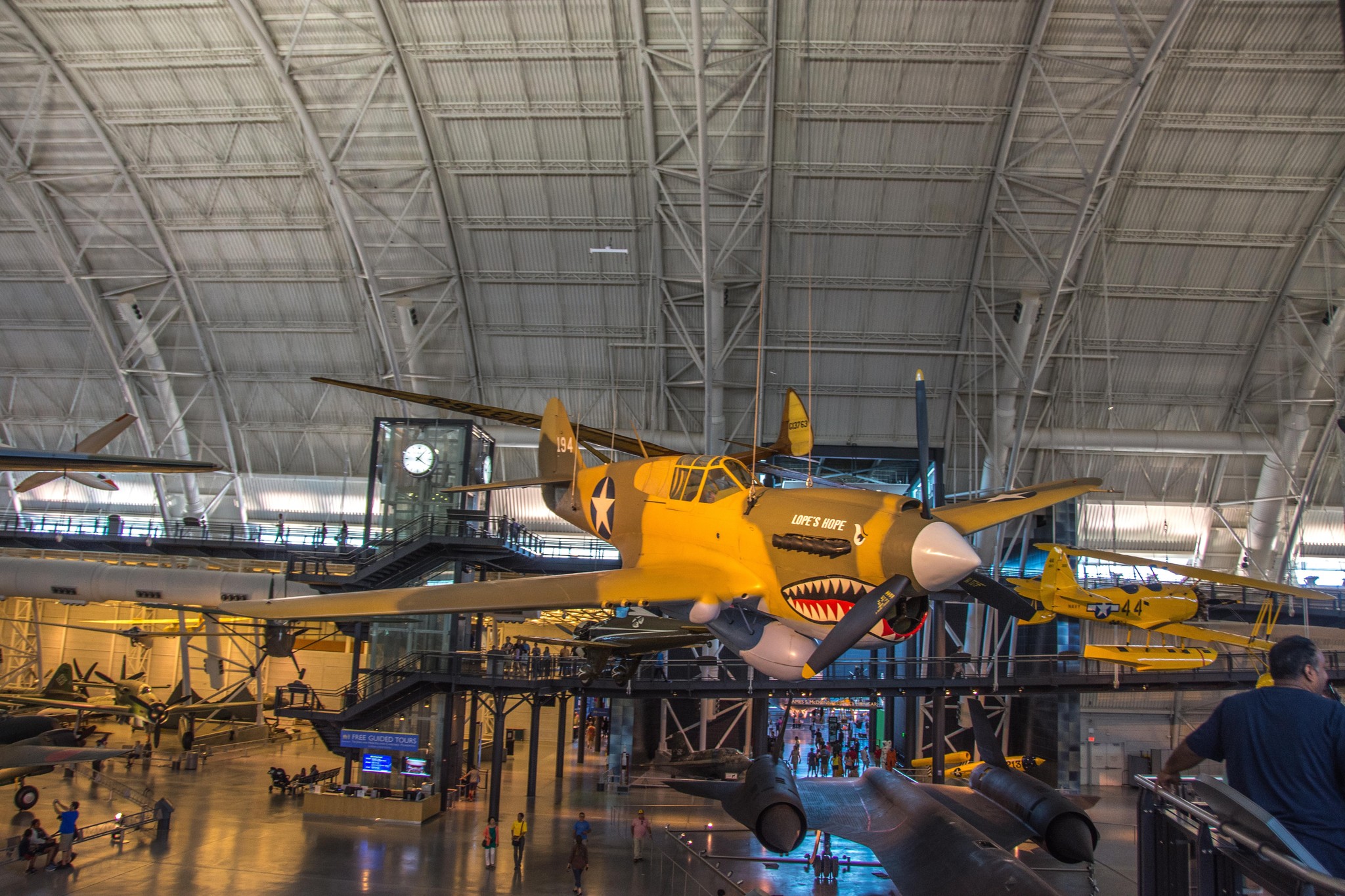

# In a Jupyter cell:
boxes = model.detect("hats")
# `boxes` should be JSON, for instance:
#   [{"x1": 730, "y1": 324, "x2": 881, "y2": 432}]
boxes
[{"x1": 638, "y1": 810, "x2": 644, "y2": 814}]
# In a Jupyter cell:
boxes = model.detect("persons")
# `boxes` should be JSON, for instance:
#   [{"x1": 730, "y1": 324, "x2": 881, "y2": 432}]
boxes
[
  {"x1": 573, "y1": 812, "x2": 591, "y2": 846},
  {"x1": 319, "y1": 520, "x2": 348, "y2": 546},
  {"x1": 1157, "y1": 635, "x2": 1345, "y2": 882},
  {"x1": 91, "y1": 726, "x2": 142, "y2": 774},
  {"x1": 181, "y1": 727, "x2": 194, "y2": 751},
  {"x1": 483, "y1": 817, "x2": 499, "y2": 870},
  {"x1": 631, "y1": 810, "x2": 652, "y2": 864},
  {"x1": 275, "y1": 514, "x2": 284, "y2": 542},
  {"x1": 653, "y1": 651, "x2": 672, "y2": 683},
  {"x1": 768, "y1": 707, "x2": 897, "y2": 777},
  {"x1": 291, "y1": 764, "x2": 319, "y2": 784},
  {"x1": 511, "y1": 813, "x2": 527, "y2": 870},
  {"x1": 567, "y1": 835, "x2": 589, "y2": 896},
  {"x1": 498, "y1": 514, "x2": 518, "y2": 543},
  {"x1": 459, "y1": 765, "x2": 480, "y2": 801},
  {"x1": 574, "y1": 711, "x2": 609, "y2": 748},
  {"x1": 491, "y1": 636, "x2": 585, "y2": 676},
  {"x1": 18, "y1": 799, "x2": 79, "y2": 873},
  {"x1": 952, "y1": 646, "x2": 965, "y2": 680}
]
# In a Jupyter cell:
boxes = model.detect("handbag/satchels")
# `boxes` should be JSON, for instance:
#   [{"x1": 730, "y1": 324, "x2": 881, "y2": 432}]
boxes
[
  {"x1": 481, "y1": 839, "x2": 486, "y2": 847},
  {"x1": 512, "y1": 840, "x2": 519, "y2": 846}
]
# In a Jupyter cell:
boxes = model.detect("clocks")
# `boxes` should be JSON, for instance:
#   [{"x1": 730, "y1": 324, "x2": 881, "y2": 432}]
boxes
[
  {"x1": 475, "y1": 452, "x2": 493, "y2": 483},
  {"x1": 400, "y1": 441, "x2": 439, "y2": 478}
]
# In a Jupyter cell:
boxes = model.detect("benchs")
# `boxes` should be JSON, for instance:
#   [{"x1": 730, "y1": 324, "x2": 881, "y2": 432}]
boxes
[{"x1": 286, "y1": 767, "x2": 342, "y2": 794}]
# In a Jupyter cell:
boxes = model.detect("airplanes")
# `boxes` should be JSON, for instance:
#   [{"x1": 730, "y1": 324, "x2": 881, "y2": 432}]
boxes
[
  {"x1": 620, "y1": 693, "x2": 1104, "y2": 896},
  {"x1": 1004, "y1": 542, "x2": 1341, "y2": 701},
  {"x1": 308, "y1": 373, "x2": 1125, "y2": 681},
  {"x1": 0, "y1": 413, "x2": 323, "y2": 812}
]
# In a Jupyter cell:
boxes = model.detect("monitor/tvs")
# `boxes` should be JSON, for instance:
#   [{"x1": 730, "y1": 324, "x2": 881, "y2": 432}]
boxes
[
  {"x1": 344, "y1": 786, "x2": 362, "y2": 797},
  {"x1": 402, "y1": 791, "x2": 416, "y2": 801},
  {"x1": 362, "y1": 754, "x2": 392, "y2": 773},
  {"x1": 400, "y1": 756, "x2": 431, "y2": 776},
  {"x1": 373, "y1": 788, "x2": 390, "y2": 798}
]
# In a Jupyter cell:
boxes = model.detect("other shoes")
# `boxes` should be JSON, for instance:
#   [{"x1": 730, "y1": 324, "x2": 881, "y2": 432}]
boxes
[
  {"x1": 633, "y1": 858, "x2": 644, "y2": 863},
  {"x1": 514, "y1": 864, "x2": 521, "y2": 870},
  {"x1": 468, "y1": 796, "x2": 473, "y2": 801},
  {"x1": 577, "y1": 892, "x2": 582, "y2": 896},
  {"x1": 573, "y1": 890, "x2": 578, "y2": 893},
  {"x1": 25, "y1": 868, "x2": 38, "y2": 875},
  {"x1": 56, "y1": 861, "x2": 71, "y2": 869},
  {"x1": 43, "y1": 863, "x2": 59, "y2": 872},
  {"x1": 123, "y1": 761, "x2": 133, "y2": 767},
  {"x1": 486, "y1": 865, "x2": 495, "y2": 869}
]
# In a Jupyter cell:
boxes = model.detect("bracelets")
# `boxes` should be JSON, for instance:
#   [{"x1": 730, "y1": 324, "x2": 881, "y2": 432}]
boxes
[{"x1": 649, "y1": 834, "x2": 652, "y2": 835}]
